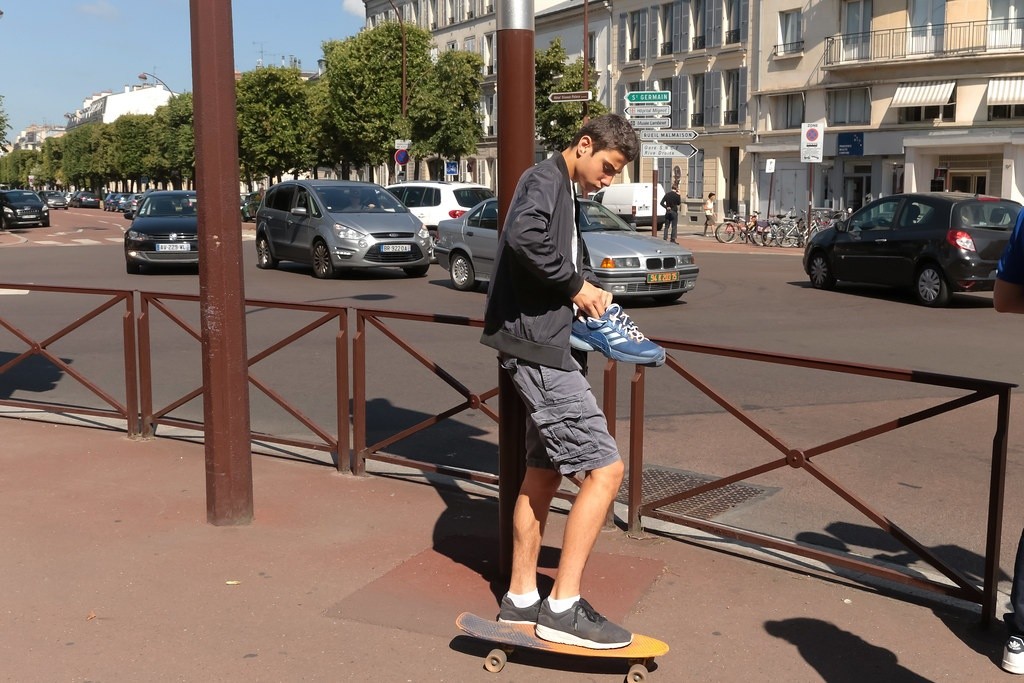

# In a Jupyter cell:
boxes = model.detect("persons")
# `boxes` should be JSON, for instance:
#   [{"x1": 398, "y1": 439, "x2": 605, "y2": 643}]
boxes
[
  {"x1": 703, "y1": 193, "x2": 721, "y2": 237},
  {"x1": 993, "y1": 204, "x2": 1024, "y2": 675},
  {"x1": 478, "y1": 114, "x2": 633, "y2": 650},
  {"x1": 660, "y1": 183, "x2": 681, "y2": 245}
]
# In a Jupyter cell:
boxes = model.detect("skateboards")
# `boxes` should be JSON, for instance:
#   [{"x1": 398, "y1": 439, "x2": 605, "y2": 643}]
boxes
[{"x1": 455, "y1": 609, "x2": 670, "y2": 683}]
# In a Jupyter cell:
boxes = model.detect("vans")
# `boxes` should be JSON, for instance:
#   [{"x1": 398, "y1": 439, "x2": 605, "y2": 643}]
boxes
[{"x1": 587, "y1": 183, "x2": 667, "y2": 230}]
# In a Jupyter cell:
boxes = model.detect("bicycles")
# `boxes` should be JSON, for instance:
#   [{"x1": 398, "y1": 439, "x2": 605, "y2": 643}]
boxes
[{"x1": 714, "y1": 206, "x2": 832, "y2": 248}]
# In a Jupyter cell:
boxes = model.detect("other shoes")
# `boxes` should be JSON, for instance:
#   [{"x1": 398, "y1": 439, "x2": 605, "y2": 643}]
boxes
[
  {"x1": 673, "y1": 241, "x2": 679, "y2": 245},
  {"x1": 704, "y1": 234, "x2": 709, "y2": 237},
  {"x1": 711, "y1": 233, "x2": 718, "y2": 237}
]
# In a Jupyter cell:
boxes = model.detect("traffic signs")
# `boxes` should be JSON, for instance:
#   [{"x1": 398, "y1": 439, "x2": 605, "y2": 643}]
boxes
[
  {"x1": 548, "y1": 91, "x2": 589, "y2": 102},
  {"x1": 641, "y1": 143, "x2": 699, "y2": 159},
  {"x1": 640, "y1": 130, "x2": 699, "y2": 141},
  {"x1": 624, "y1": 105, "x2": 671, "y2": 116},
  {"x1": 627, "y1": 118, "x2": 671, "y2": 128},
  {"x1": 624, "y1": 91, "x2": 671, "y2": 104}
]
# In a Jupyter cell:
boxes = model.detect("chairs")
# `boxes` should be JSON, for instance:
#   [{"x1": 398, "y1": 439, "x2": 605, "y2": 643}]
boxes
[
  {"x1": 154, "y1": 200, "x2": 173, "y2": 215},
  {"x1": 481, "y1": 207, "x2": 498, "y2": 230}
]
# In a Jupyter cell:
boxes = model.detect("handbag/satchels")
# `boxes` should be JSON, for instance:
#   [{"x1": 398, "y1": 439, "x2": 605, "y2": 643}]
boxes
[{"x1": 665, "y1": 210, "x2": 678, "y2": 220}]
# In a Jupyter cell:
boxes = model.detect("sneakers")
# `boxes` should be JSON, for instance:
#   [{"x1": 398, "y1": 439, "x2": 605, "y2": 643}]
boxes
[
  {"x1": 1002, "y1": 636, "x2": 1024, "y2": 674},
  {"x1": 497, "y1": 593, "x2": 541, "y2": 624},
  {"x1": 537, "y1": 596, "x2": 633, "y2": 651},
  {"x1": 566, "y1": 305, "x2": 661, "y2": 363}
]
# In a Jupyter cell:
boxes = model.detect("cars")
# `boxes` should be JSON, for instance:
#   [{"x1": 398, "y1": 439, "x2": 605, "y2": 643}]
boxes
[
  {"x1": 111, "y1": 193, "x2": 130, "y2": 212},
  {"x1": 137, "y1": 189, "x2": 163, "y2": 214},
  {"x1": 103, "y1": 193, "x2": 118, "y2": 211},
  {"x1": 376, "y1": 181, "x2": 498, "y2": 265},
  {"x1": 241, "y1": 179, "x2": 433, "y2": 280},
  {"x1": 123, "y1": 191, "x2": 199, "y2": 275},
  {"x1": 60, "y1": 191, "x2": 100, "y2": 208},
  {"x1": 802, "y1": 192, "x2": 1024, "y2": 309},
  {"x1": 38, "y1": 191, "x2": 68, "y2": 210},
  {"x1": 433, "y1": 197, "x2": 699, "y2": 303},
  {"x1": 0, "y1": 190, "x2": 50, "y2": 229},
  {"x1": 124, "y1": 193, "x2": 143, "y2": 213}
]
